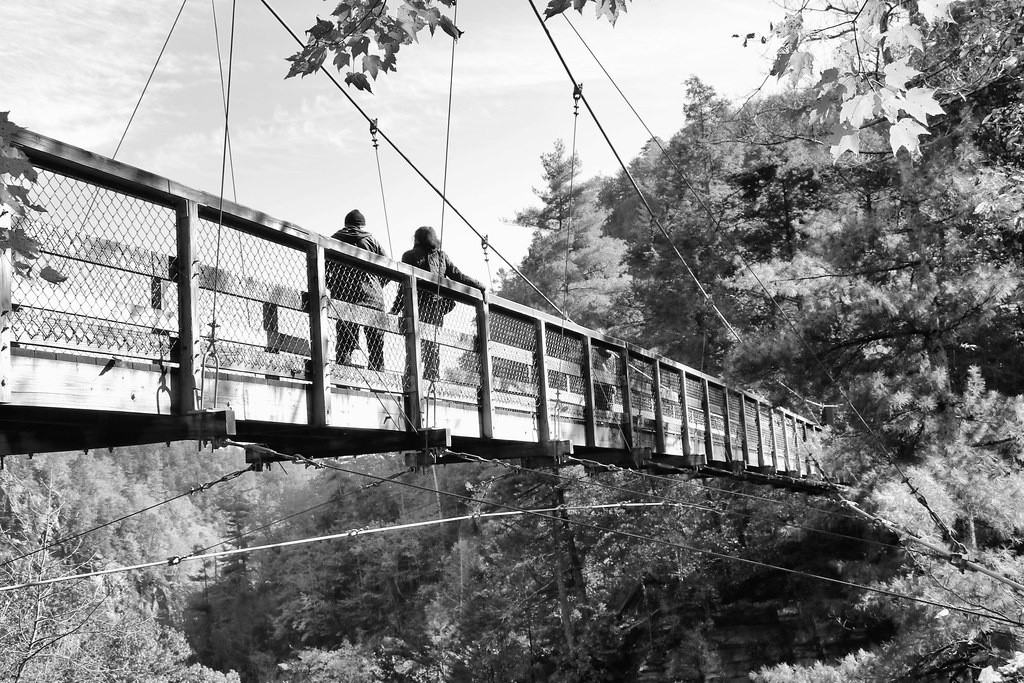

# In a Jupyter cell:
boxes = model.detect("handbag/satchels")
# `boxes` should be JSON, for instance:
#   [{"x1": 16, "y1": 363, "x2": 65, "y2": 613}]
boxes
[{"x1": 433, "y1": 250, "x2": 456, "y2": 319}]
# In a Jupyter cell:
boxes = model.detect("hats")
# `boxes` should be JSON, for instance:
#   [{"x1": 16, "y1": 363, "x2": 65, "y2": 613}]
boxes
[{"x1": 344, "y1": 209, "x2": 366, "y2": 226}]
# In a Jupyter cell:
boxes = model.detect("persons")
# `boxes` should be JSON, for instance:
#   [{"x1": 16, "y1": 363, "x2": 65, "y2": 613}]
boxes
[
  {"x1": 591, "y1": 345, "x2": 617, "y2": 410},
  {"x1": 324, "y1": 209, "x2": 392, "y2": 392},
  {"x1": 388, "y1": 226, "x2": 486, "y2": 383}
]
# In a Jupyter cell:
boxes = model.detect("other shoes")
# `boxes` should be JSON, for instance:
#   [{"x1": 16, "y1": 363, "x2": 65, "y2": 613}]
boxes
[{"x1": 423, "y1": 371, "x2": 441, "y2": 382}]
